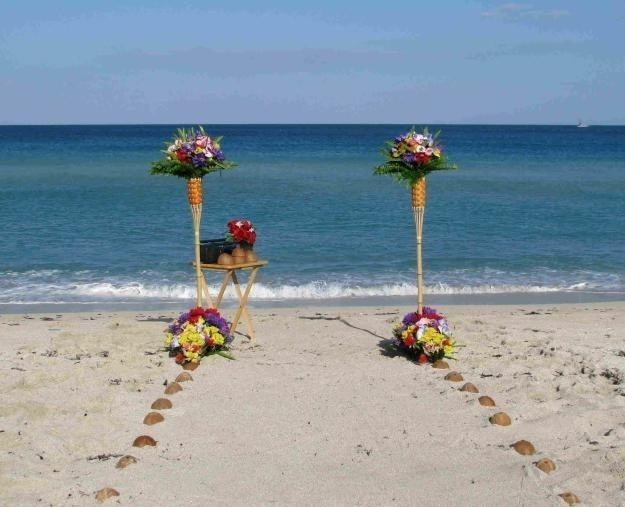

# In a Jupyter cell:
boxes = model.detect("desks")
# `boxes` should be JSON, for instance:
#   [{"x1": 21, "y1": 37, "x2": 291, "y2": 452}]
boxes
[{"x1": 193, "y1": 259, "x2": 269, "y2": 339}]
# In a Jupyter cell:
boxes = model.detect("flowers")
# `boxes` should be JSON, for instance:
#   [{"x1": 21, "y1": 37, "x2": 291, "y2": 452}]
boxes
[
  {"x1": 162, "y1": 304, "x2": 248, "y2": 362},
  {"x1": 223, "y1": 219, "x2": 257, "y2": 251},
  {"x1": 148, "y1": 122, "x2": 239, "y2": 179},
  {"x1": 392, "y1": 305, "x2": 468, "y2": 363},
  {"x1": 372, "y1": 124, "x2": 457, "y2": 190}
]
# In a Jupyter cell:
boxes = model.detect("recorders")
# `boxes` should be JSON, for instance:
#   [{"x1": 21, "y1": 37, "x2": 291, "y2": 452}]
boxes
[{"x1": 199, "y1": 237, "x2": 238, "y2": 264}]
